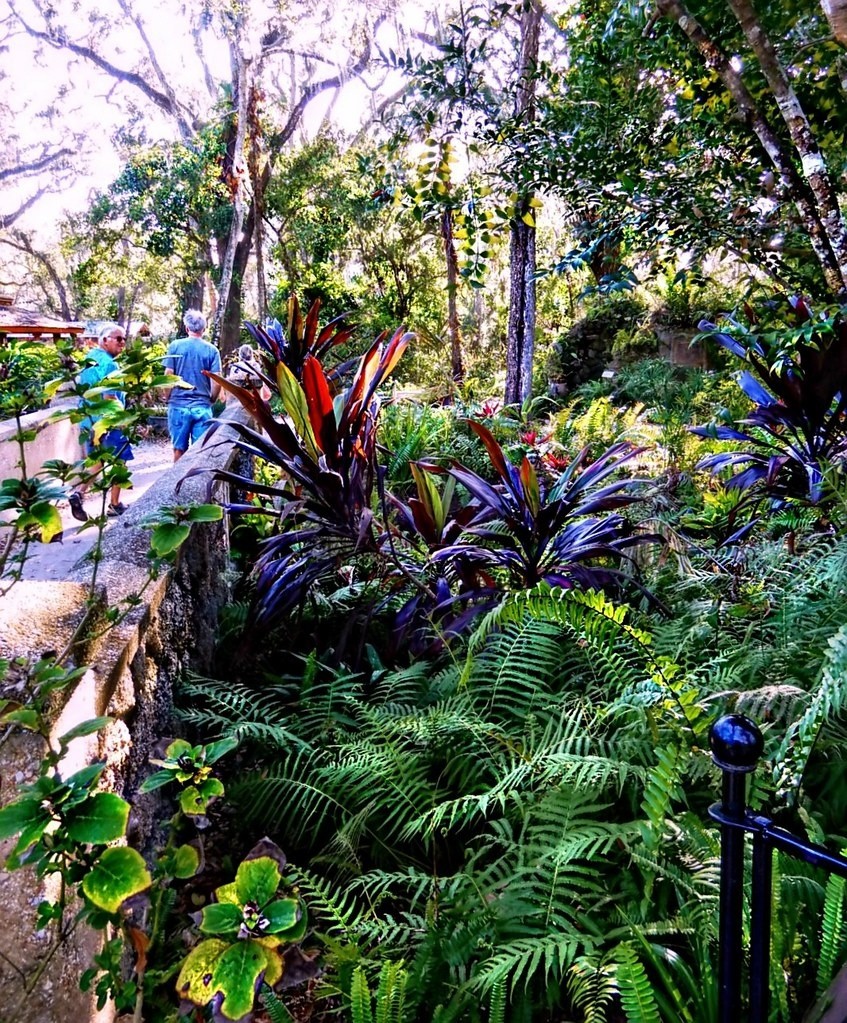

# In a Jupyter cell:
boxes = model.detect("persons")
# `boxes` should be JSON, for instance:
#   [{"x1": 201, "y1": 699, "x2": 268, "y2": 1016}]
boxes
[
  {"x1": 161, "y1": 308, "x2": 222, "y2": 464},
  {"x1": 69, "y1": 324, "x2": 135, "y2": 522},
  {"x1": 230, "y1": 344, "x2": 262, "y2": 376}
]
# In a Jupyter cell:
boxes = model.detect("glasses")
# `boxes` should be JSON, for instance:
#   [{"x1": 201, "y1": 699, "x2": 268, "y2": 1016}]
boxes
[{"x1": 114, "y1": 336, "x2": 126, "y2": 342}]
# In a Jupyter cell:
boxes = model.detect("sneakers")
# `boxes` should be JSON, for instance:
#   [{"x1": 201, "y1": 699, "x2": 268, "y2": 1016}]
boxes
[
  {"x1": 107, "y1": 502, "x2": 129, "y2": 516},
  {"x1": 68, "y1": 494, "x2": 88, "y2": 522}
]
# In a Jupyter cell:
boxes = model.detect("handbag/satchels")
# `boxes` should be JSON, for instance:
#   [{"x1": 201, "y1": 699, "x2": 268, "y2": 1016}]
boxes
[{"x1": 260, "y1": 383, "x2": 272, "y2": 400}]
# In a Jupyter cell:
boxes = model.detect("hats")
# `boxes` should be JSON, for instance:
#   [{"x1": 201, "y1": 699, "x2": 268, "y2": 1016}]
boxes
[{"x1": 137, "y1": 324, "x2": 149, "y2": 332}]
